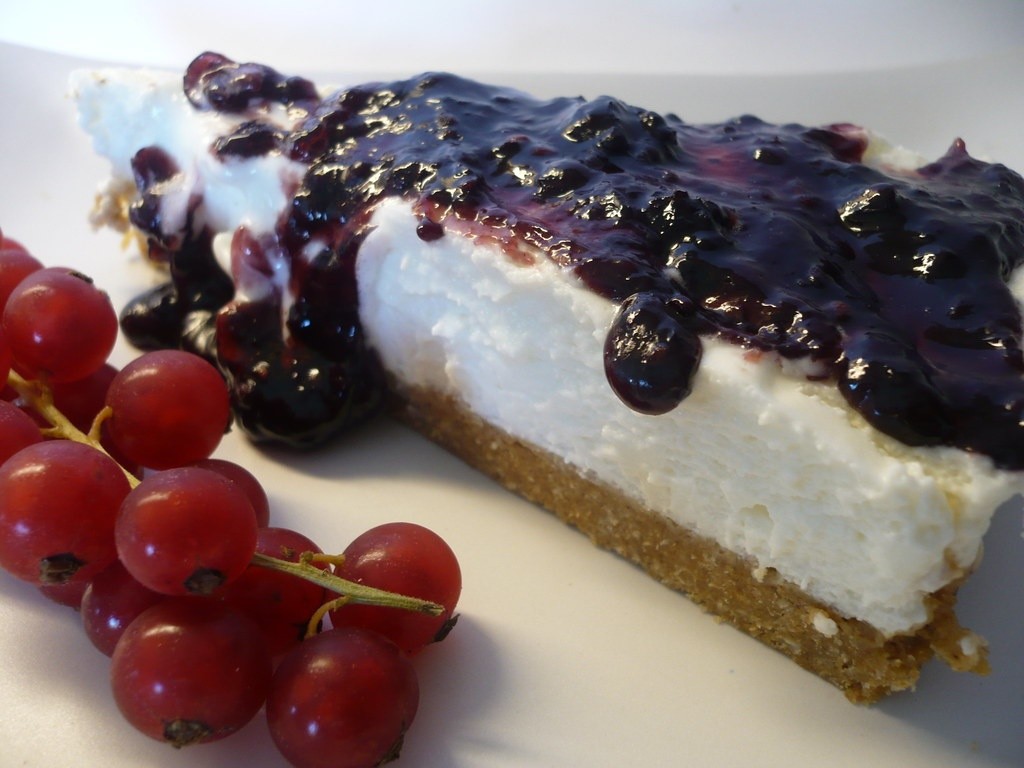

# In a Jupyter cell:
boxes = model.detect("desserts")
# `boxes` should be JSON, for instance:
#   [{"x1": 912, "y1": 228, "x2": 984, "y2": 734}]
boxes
[{"x1": 67, "y1": 50, "x2": 1024, "y2": 705}]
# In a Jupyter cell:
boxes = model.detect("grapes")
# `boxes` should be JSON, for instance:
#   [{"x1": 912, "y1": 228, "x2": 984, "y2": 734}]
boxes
[{"x1": 0, "y1": 230, "x2": 463, "y2": 768}]
